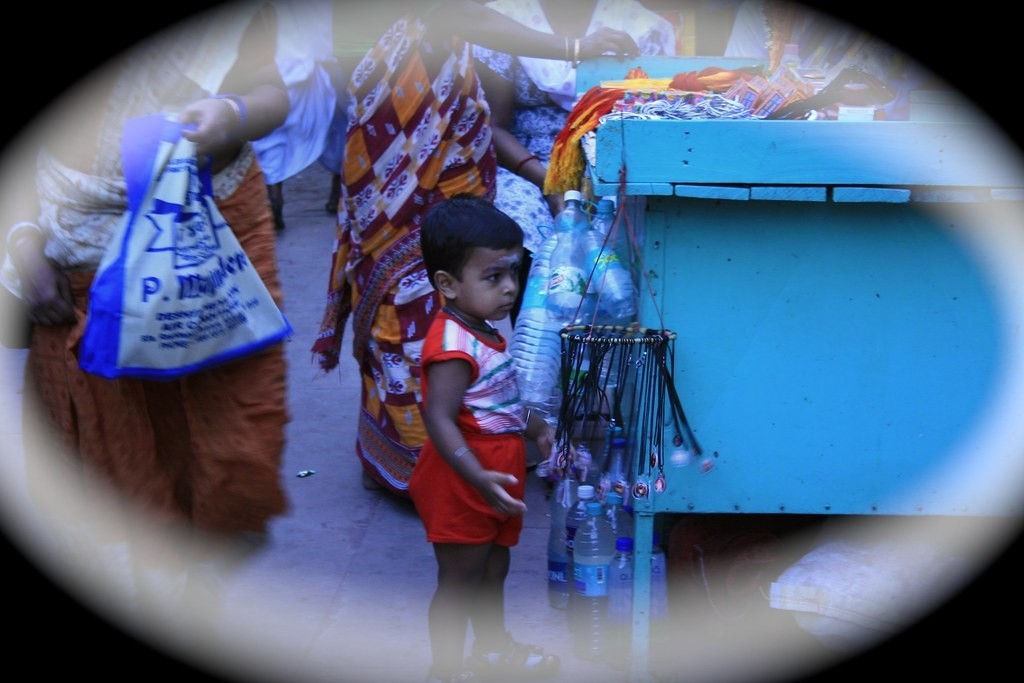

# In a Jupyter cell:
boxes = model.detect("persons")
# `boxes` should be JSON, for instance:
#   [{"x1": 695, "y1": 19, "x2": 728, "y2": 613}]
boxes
[
  {"x1": 722, "y1": 0, "x2": 934, "y2": 121},
  {"x1": 309, "y1": 0, "x2": 642, "y2": 522},
  {"x1": 407, "y1": 192, "x2": 560, "y2": 681},
  {"x1": 0, "y1": 5, "x2": 299, "y2": 570},
  {"x1": 469, "y1": 0, "x2": 676, "y2": 429}
]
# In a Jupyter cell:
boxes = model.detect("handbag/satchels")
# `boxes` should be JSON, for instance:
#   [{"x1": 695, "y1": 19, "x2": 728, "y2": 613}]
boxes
[{"x1": 79, "y1": 94, "x2": 294, "y2": 379}]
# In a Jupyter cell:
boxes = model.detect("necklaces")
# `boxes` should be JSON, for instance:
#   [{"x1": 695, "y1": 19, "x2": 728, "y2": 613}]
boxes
[{"x1": 443, "y1": 305, "x2": 499, "y2": 336}]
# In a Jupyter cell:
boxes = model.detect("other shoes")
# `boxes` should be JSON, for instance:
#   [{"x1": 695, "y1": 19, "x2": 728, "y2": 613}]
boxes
[{"x1": 425, "y1": 632, "x2": 559, "y2": 682}]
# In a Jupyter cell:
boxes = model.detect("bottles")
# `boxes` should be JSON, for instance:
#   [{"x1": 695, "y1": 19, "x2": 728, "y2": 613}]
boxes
[
  {"x1": 645, "y1": 531, "x2": 668, "y2": 623},
  {"x1": 506, "y1": 186, "x2": 641, "y2": 484},
  {"x1": 563, "y1": 486, "x2": 598, "y2": 615},
  {"x1": 546, "y1": 464, "x2": 582, "y2": 611},
  {"x1": 601, "y1": 491, "x2": 632, "y2": 543},
  {"x1": 602, "y1": 536, "x2": 633, "y2": 643},
  {"x1": 778, "y1": 43, "x2": 802, "y2": 90},
  {"x1": 572, "y1": 501, "x2": 612, "y2": 659}
]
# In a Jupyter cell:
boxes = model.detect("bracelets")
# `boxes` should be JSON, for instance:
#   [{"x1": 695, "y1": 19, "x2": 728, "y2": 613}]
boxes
[
  {"x1": 565, "y1": 36, "x2": 569, "y2": 62},
  {"x1": 571, "y1": 38, "x2": 583, "y2": 69}
]
